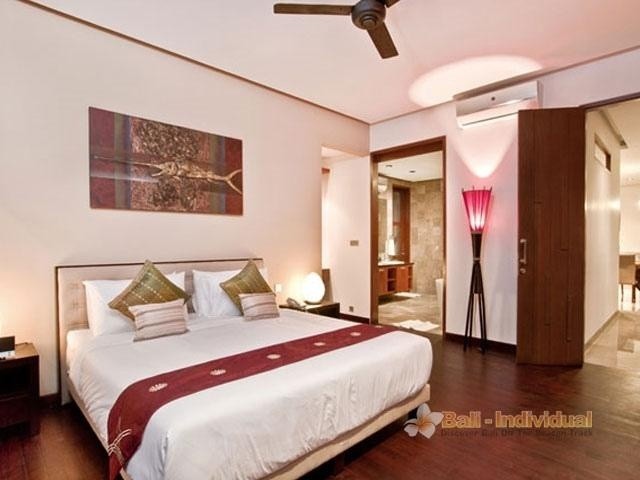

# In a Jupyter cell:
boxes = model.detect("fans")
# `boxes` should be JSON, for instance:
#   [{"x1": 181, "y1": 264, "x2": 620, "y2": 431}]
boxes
[{"x1": 274, "y1": 0, "x2": 400, "y2": 58}]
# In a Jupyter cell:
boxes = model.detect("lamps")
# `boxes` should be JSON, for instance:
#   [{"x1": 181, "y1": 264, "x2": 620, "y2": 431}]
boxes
[
  {"x1": 303, "y1": 272, "x2": 326, "y2": 306},
  {"x1": 461, "y1": 187, "x2": 492, "y2": 352}
]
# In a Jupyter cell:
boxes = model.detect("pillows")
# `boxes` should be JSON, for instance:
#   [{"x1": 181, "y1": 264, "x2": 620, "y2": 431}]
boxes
[
  {"x1": 221, "y1": 258, "x2": 276, "y2": 315},
  {"x1": 108, "y1": 259, "x2": 191, "y2": 321},
  {"x1": 82, "y1": 272, "x2": 186, "y2": 337},
  {"x1": 128, "y1": 299, "x2": 189, "y2": 342},
  {"x1": 240, "y1": 292, "x2": 280, "y2": 320},
  {"x1": 192, "y1": 267, "x2": 269, "y2": 317}
]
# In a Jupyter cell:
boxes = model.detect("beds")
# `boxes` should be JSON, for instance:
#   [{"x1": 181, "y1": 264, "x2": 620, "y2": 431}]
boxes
[{"x1": 54, "y1": 259, "x2": 433, "y2": 480}]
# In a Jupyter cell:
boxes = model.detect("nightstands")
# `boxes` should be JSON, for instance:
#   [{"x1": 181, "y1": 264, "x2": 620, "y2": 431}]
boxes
[
  {"x1": 0, "y1": 342, "x2": 42, "y2": 435},
  {"x1": 279, "y1": 302, "x2": 340, "y2": 319}
]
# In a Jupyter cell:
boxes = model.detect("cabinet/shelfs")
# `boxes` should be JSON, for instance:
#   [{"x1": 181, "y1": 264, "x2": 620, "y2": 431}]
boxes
[{"x1": 378, "y1": 262, "x2": 414, "y2": 301}]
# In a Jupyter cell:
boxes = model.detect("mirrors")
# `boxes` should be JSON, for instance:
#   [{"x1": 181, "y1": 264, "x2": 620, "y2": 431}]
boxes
[{"x1": 379, "y1": 198, "x2": 387, "y2": 254}]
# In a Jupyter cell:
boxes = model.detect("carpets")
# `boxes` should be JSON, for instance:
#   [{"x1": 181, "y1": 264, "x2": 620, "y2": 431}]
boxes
[{"x1": 379, "y1": 295, "x2": 441, "y2": 331}]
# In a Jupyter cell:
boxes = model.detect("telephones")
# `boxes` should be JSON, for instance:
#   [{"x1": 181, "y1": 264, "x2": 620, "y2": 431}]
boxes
[{"x1": 287, "y1": 296, "x2": 307, "y2": 309}]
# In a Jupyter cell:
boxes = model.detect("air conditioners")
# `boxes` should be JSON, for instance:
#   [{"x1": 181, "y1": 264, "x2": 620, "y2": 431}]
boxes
[{"x1": 456, "y1": 81, "x2": 543, "y2": 128}]
